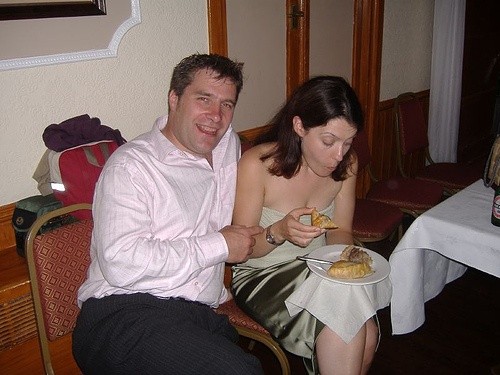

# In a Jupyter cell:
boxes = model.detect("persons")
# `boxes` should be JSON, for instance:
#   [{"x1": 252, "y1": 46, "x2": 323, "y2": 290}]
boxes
[
  {"x1": 71, "y1": 53, "x2": 264, "y2": 375},
  {"x1": 231, "y1": 75, "x2": 380, "y2": 375}
]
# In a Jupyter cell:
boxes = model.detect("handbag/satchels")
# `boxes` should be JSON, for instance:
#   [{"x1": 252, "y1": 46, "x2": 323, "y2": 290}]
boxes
[
  {"x1": 48, "y1": 140, "x2": 127, "y2": 220},
  {"x1": 12, "y1": 195, "x2": 64, "y2": 258},
  {"x1": 484, "y1": 134, "x2": 500, "y2": 188}
]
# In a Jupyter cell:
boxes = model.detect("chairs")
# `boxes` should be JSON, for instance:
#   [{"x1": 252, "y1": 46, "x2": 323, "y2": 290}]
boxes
[
  {"x1": 26, "y1": 203, "x2": 94, "y2": 375},
  {"x1": 352, "y1": 91, "x2": 485, "y2": 248},
  {"x1": 216, "y1": 263, "x2": 291, "y2": 375}
]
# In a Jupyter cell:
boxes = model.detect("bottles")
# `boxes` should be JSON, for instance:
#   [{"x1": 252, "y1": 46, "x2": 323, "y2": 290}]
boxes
[{"x1": 491, "y1": 183, "x2": 500, "y2": 227}]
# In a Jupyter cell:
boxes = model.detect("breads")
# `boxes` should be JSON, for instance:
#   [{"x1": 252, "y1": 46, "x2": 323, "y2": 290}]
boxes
[
  {"x1": 310, "y1": 210, "x2": 339, "y2": 229},
  {"x1": 328, "y1": 260, "x2": 374, "y2": 279}
]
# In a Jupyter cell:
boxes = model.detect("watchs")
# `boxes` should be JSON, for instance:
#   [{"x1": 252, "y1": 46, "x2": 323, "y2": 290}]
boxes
[{"x1": 265, "y1": 225, "x2": 286, "y2": 247}]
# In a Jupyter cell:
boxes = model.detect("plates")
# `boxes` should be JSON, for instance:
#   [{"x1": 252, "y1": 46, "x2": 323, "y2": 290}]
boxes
[{"x1": 305, "y1": 244, "x2": 391, "y2": 285}]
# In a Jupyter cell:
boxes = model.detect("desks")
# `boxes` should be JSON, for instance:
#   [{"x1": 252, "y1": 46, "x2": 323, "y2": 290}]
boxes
[{"x1": 389, "y1": 178, "x2": 500, "y2": 335}]
